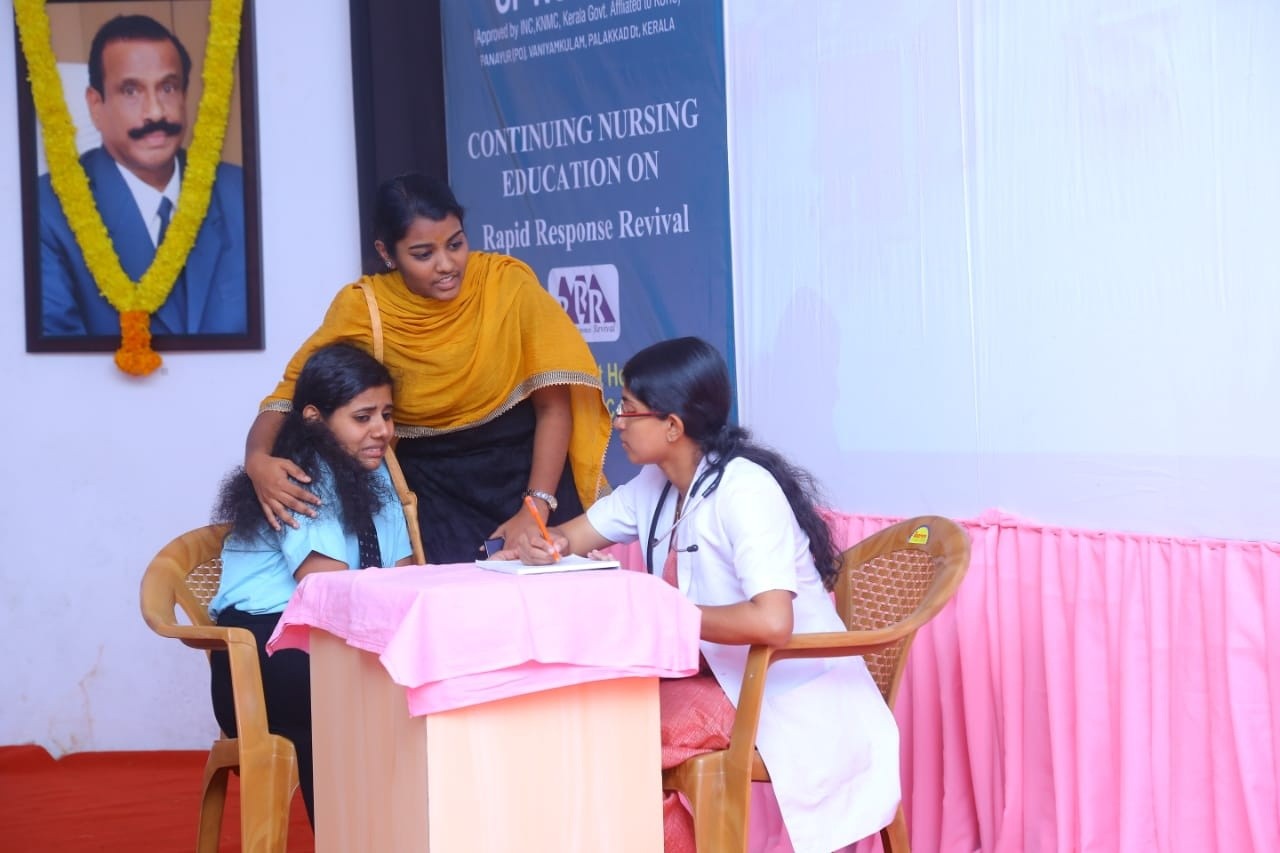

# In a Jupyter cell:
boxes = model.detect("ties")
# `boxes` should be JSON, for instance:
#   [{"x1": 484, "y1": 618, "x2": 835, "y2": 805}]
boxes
[
  {"x1": 356, "y1": 518, "x2": 383, "y2": 568},
  {"x1": 154, "y1": 195, "x2": 188, "y2": 335}
]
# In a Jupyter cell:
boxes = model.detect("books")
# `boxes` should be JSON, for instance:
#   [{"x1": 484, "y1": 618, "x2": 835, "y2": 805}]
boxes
[{"x1": 475, "y1": 554, "x2": 619, "y2": 575}]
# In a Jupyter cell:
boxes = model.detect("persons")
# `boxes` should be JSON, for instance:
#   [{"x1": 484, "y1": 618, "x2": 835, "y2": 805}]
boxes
[
  {"x1": 208, "y1": 343, "x2": 414, "y2": 834},
  {"x1": 517, "y1": 337, "x2": 902, "y2": 853},
  {"x1": 244, "y1": 174, "x2": 613, "y2": 565},
  {"x1": 38, "y1": 15, "x2": 247, "y2": 334}
]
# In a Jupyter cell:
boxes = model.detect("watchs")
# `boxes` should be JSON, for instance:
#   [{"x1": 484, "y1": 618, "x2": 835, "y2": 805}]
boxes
[{"x1": 521, "y1": 489, "x2": 557, "y2": 512}]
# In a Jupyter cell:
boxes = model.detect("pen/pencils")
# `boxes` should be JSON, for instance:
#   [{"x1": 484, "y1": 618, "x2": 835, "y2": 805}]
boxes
[{"x1": 521, "y1": 490, "x2": 562, "y2": 565}]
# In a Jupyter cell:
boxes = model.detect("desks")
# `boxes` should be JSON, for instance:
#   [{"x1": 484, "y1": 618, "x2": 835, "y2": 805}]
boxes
[{"x1": 264, "y1": 559, "x2": 701, "y2": 853}]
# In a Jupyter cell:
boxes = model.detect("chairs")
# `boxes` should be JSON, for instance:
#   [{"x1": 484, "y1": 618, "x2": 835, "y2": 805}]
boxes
[
  {"x1": 142, "y1": 523, "x2": 306, "y2": 853},
  {"x1": 661, "y1": 514, "x2": 973, "y2": 853}
]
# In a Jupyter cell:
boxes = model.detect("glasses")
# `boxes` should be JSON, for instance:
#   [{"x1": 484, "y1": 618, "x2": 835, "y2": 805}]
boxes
[{"x1": 615, "y1": 399, "x2": 669, "y2": 424}]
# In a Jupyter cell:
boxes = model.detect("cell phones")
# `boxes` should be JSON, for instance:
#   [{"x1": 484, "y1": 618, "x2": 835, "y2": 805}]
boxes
[{"x1": 484, "y1": 538, "x2": 504, "y2": 559}]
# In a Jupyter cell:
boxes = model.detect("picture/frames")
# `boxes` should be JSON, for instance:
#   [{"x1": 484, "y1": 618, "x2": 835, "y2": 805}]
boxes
[{"x1": 13, "y1": 0, "x2": 265, "y2": 355}]
